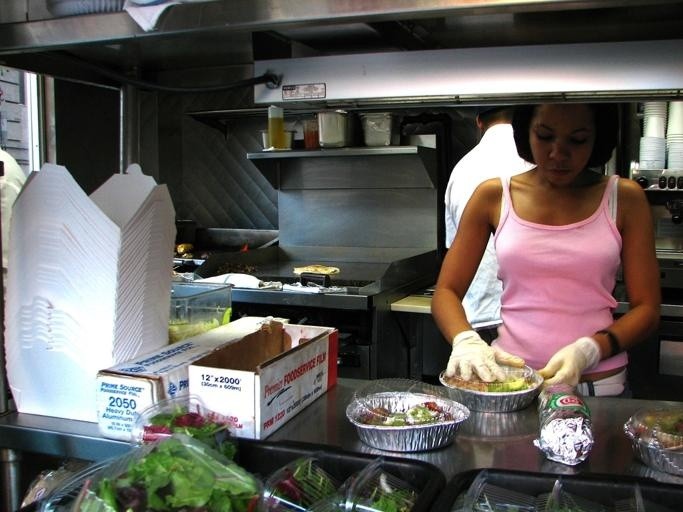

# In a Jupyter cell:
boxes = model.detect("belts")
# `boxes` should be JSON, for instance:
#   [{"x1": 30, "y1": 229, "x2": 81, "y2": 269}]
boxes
[{"x1": 577, "y1": 367, "x2": 627, "y2": 397}]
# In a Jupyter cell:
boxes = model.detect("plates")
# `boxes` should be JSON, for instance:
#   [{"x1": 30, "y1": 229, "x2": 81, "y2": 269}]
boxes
[
  {"x1": 344, "y1": 391, "x2": 462, "y2": 453},
  {"x1": 436, "y1": 370, "x2": 543, "y2": 411},
  {"x1": 622, "y1": 406, "x2": 682, "y2": 475}
]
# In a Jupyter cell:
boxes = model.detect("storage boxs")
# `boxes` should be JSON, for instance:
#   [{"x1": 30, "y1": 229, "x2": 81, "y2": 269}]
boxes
[
  {"x1": 186, "y1": 320, "x2": 341, "y2": 445},
  {"x1": 94, "y1": 316, "x2": 290, "y2": 439}
]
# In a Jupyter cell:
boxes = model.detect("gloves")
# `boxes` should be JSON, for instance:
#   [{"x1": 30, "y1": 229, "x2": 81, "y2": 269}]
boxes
[
  {"x1": 445, "y1": 330, "x2": 525, "y2": 383},
  {"x1": 536, "y1": 336, "x2": 600, "y2": 391}
]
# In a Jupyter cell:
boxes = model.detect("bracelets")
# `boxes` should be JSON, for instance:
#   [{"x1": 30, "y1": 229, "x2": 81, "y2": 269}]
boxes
[{"x1": 594, "y1": 327, "x2": 622, "y2": 358}]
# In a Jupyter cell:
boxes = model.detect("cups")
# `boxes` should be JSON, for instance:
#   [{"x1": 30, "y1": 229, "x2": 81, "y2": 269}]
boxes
[
  {"x1": 637, "y1": 101, "x2": 683, "y2": 172},
  {"x1": 302, "y1": 118, "x2": 318, "y2": 150}
]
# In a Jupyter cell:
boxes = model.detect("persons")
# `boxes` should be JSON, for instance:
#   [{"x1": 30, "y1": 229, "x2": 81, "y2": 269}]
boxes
[
  {"x1": 440, "y1": 104, "x2": 537, "y2": 343},
  {"x1": 428, "y1": 102, "x2": 662, "y2": 402}
]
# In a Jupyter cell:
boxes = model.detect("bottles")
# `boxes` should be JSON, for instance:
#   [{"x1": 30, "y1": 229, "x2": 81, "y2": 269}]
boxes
[{"x1": 266, "y1": 104, "x2": 284, "y2": 149}]
